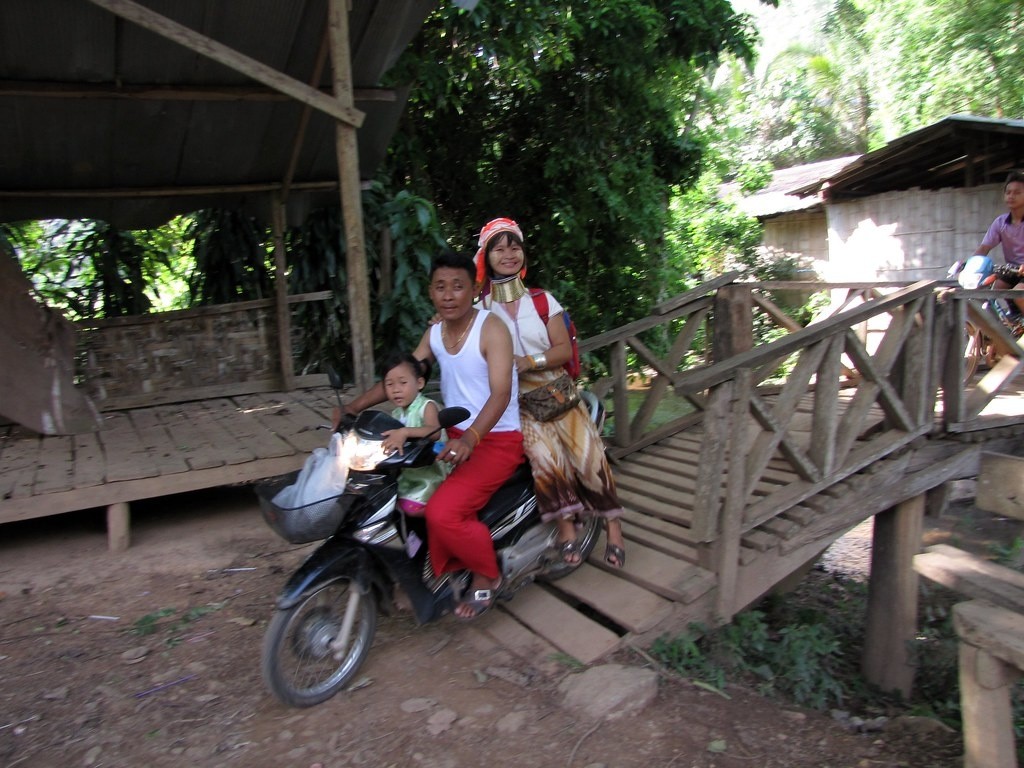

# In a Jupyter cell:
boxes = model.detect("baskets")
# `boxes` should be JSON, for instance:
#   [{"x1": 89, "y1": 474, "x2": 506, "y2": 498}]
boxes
[{"x1": 260, "y1": 482, "x2": 353, "y2": 544}]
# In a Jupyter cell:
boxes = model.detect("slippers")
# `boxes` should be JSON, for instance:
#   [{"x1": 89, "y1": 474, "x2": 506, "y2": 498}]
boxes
[
  {"x1": 604, "y1": 543, "x2": 626, "y2": 568},
  {"x1": 556, "y1": 536, "x2": 582, "y2": 567},
  {"x1": 456, "y1": 574, "x2": 507, "y2": 621}
]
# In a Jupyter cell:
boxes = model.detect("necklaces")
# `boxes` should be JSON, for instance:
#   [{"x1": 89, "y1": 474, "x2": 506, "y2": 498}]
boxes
[
  {"x1": 489, "y1": 275, "x2": 527, "y2": 303},
  {"x1": 444, "y1": 308, "x2": 475, "y2": 349}
]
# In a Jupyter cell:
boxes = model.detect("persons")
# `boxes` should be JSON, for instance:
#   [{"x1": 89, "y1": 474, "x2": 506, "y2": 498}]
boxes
[
  {"x1": 381, "y1": 352, "x2": 455, "y2": 515},
  {"x1": 330, "y1": 257, "x2": 526, "y2": 624},
  {"x1": 427, "y1": 218, "x2": 628, "y2": 568},
  {"x1": 947, "y1": 171, "x2": 1024, "y2": 313}
]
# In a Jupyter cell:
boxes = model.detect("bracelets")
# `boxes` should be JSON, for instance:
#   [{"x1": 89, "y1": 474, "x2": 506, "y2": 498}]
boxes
[
  {"x1": 526, "y1": 352, "x2": 547, "y2": 371},
  {"x1": 467, "y1": 426, "x2": 481, "y2": 445}
]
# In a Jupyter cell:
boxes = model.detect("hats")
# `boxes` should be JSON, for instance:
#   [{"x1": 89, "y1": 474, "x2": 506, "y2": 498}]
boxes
[{"x1": 474, "y1": 218, "x2": 527, "y2": 280}]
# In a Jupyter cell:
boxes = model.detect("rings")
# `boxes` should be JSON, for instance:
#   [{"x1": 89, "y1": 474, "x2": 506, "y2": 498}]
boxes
[{"x1": 449, "y1": 450, "x2": 456, "y2": 455}]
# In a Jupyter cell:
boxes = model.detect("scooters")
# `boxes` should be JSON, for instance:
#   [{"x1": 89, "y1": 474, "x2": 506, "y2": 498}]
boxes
[
  {"x1": 260, "y1": 369, "x2": 606, "y2": 707},
  {"x1": 945, "y1": 256, "x2": 1024, "y2": 382}
]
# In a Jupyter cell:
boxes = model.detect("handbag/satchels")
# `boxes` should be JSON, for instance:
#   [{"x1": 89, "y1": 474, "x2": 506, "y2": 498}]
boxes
[
  {"x1": 269, "y1": 432, "x2": 350, "y2": 532},
  {"x1": 521, "y1": 373, "x2": 581, "y2": 423}
]
